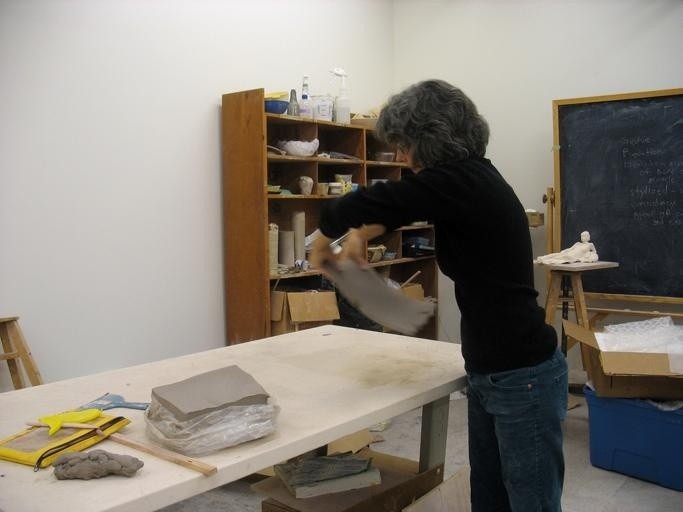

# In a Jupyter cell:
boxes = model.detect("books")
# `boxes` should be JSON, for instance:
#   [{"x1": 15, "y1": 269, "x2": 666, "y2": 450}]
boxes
[{"x1": 273, "y1": 462, "x2": 382, "y2": 500}]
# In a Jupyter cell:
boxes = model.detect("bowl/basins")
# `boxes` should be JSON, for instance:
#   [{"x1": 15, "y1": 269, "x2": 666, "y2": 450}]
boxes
[
  {"x1": 349, "y1": 118, "x2": 377, "y2": 128},
  {"x1": 373, "y1": 152, "x2": 395, "y2": 162},
  {"x1": 367, "y1": 243, "x2": 397, "y2": 262},
  {"x1": 263, "y1": 89, "x2": 289, "y2": 114},
  {"x1": 267, "y1": 138, "x2": 321, "y2": 157},
  {"x1": 411, "y1": 221, "x2": 428, "y2": 227},
  {"x1": 369, "y1": 179, "x2": 389, "y2": 186}
]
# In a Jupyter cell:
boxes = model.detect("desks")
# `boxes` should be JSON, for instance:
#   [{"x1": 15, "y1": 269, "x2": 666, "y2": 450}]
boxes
[
  {"x1": 1, "y1": 320, "x2": 469, "y2": 512},
  {"x1": 535, "y1": 259, "x2": 619, "y2": 401}
]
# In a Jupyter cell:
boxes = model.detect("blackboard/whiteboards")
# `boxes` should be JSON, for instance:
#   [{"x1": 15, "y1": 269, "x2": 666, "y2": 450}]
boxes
[{"x1": 552, "y1": 87, "x2": 683, "y2": 305}]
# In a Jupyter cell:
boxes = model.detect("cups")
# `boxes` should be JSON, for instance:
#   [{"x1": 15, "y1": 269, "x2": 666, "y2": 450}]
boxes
[
  {"x1": 268, "y1": 210, "x2": 309, "y2": 274},
  {"x1": 310, "y1": 96, "x2": 333, "y2": 122},
  {"x1": 525, "y1": 208, "x2": 537, "y2": 229}
]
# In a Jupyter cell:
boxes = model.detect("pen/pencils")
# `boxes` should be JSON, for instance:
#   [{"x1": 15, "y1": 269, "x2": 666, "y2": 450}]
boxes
[{"x1": 27, "y1": 420, "x2": 94, "y2": 429}]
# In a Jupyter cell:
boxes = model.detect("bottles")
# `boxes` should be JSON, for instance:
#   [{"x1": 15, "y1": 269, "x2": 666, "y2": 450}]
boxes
[
  {"x1": 287, "y1": 75, "x2": 312, "y2": 121},
  {"x1": 298, "y1": 173, "x2": 359, "y2": 196}
]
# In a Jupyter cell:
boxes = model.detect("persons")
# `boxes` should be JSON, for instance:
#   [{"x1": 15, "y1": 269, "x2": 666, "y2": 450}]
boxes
[
  {"x1": 536, "y1": 230, "x2": 598, "y2": 265},
  {"x1": 308, "y1": 78, "x2": 569, "y2": 511}
]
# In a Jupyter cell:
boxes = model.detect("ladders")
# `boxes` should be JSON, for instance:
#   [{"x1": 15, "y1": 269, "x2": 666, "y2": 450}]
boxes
[{"x1": 0, "y1": 316, "x2": 45, "y2": 394}]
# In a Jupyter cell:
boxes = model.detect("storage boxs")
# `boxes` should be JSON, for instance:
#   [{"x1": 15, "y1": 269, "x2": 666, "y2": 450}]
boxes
[
  {"x1": 561, "y1": 314, "x2": 682, "y2": 407},
  {"x1": 581, "y1": 381, "x2": 681, "y2": 492},
  {"x1": 265, "y1": 283, "x2": 340, "y2": 338},
  {"x1": 373, "y1": 280, "x2": 425, "y2": 332}
]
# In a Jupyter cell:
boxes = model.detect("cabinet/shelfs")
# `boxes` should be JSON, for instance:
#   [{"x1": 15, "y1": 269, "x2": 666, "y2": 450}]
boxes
[{"x1": 218, "y1": 89, "x2": 439, "y2": 356}]
